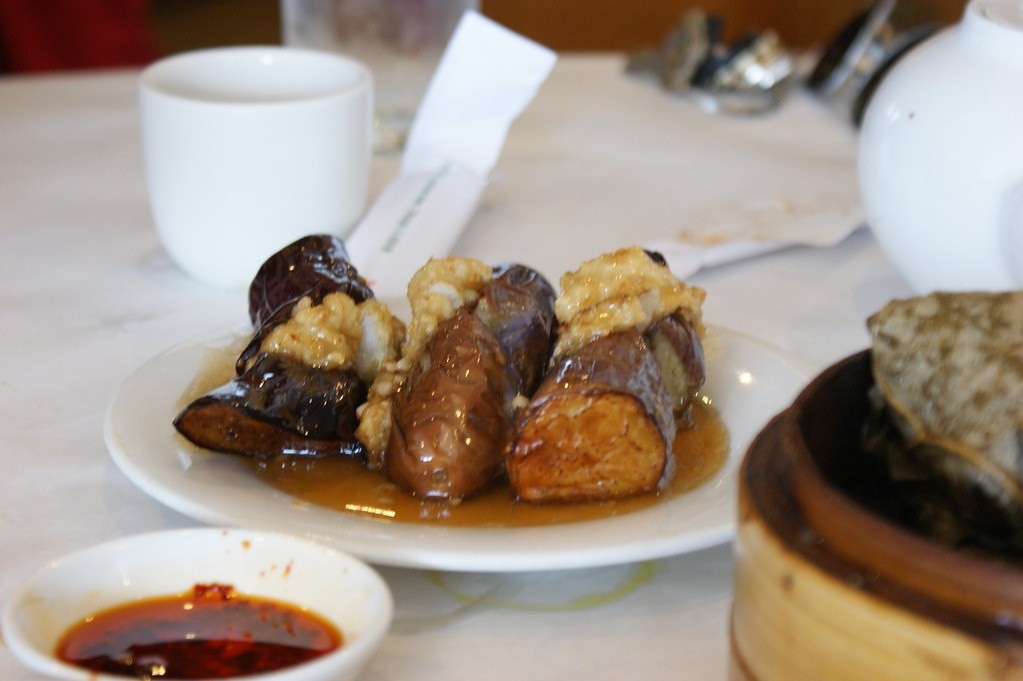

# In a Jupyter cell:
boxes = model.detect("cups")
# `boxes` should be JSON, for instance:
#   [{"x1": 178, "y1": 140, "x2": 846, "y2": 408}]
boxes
[
  {"x1": 281, "y1": 2, "x2": 480, "y2": 157},
  {"x1": 135, "y1": 46, "x2": 371, "y2": 290}
]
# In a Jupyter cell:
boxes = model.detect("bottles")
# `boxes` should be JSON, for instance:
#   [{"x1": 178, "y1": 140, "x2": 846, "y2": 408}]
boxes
[{"x1": 858, "y1": 0, "x2": 1023, "y2": 297}]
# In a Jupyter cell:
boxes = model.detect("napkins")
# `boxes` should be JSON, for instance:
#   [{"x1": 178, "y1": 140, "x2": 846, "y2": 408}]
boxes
[{"x1": 370, "y1": 57, "x2": 867, "y2": 278}]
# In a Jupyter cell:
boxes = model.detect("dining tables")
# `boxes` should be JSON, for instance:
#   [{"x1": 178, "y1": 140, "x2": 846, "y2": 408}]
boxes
[{"x1": 0, "y1": 54, "x2": 913, "y2": 681}]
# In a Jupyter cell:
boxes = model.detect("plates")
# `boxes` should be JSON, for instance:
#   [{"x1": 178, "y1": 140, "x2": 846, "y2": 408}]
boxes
[
  {"x1": 106, "y1": 292, "x2": 810, "y2": 572},
  {"x1": 0, "y1": 526, "x2": 392, "y2": 681}
]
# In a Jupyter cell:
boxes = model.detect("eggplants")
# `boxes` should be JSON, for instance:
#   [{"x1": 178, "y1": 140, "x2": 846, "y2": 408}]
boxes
[{"x1": 169, "y1": 232, "x2": 707, "y2": 504}]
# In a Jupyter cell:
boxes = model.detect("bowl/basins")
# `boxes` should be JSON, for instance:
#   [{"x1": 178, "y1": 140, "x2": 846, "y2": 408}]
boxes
[{"x1": 725, "y1": 344, "x2": 1023, "y2": 681}]
